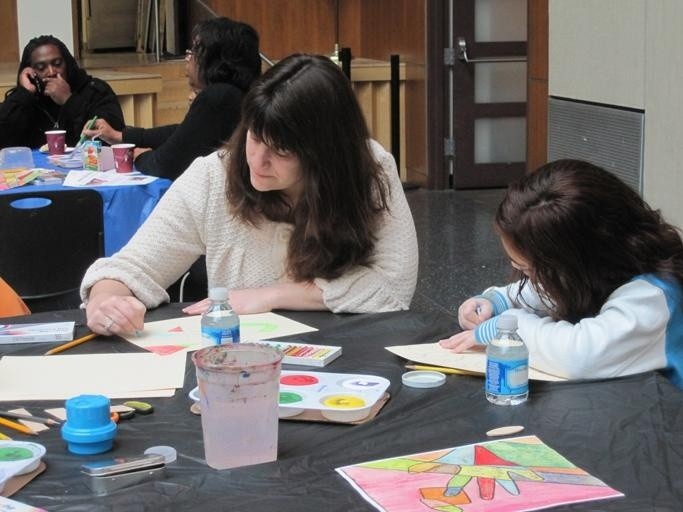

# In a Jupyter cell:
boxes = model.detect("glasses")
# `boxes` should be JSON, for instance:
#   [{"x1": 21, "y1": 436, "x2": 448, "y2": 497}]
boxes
[{"x1": 184, "y1": 49, "x2": 195, "y2": 61}]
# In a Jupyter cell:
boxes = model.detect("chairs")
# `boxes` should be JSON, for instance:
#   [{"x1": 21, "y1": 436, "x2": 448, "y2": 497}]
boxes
[{"x1": 0, "y1": 189, "x2": 105, "y2": 315}]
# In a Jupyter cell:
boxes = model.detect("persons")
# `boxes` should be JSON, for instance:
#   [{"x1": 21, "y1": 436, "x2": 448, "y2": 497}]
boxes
[
  {"x1": 80, "y1": 53, "x2": 419, "y2": 338},
  {"x1": 1, "y1": 35, "x2": 126, "y2": 146},
  {"x1": 83, "y1": 16, "x2": 261, "y2": 182},
  {"x1": 188, "y1": 87, "x2": 202, "y2": 106},
  {"x1": 438, "y1": 158, "x2": 683, "y2": 392}
]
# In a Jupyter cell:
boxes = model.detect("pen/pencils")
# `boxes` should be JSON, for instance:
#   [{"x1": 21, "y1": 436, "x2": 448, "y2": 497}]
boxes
[
  {"x1": 405, "y1": 365, "x2": 485, "y2": 377},
  {"x1": 44, "y1": 334, "x2": 97, "y2": 356},
  {"x1": 76, "y1": 115, "x2": 98, "y2": 147},
  {"x1": 0, "y1": 410, "x2": 61, "y2": 425},
  {"x1": 0, "y1": 417, "x2": 40, "y2": 436},
  {"x1": 0, "y1": 432, "x2": 14, "y2": 441}
]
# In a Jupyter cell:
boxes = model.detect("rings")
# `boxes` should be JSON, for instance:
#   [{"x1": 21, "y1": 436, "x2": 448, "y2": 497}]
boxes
[{"x1": 104, "y1": 321, "x2": 114, "y2": 330}]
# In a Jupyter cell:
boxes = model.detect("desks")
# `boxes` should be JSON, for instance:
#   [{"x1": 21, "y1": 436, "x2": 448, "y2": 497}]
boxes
[
  {"x1": 0, "y1": 302, "x2": 681, "y2": 512},
  {"x1": 0, "y1": 146, "x2": 173, "y2": 258}
]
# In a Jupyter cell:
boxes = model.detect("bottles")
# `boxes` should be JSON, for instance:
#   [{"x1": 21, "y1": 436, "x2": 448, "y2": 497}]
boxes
[
  {"x1": 486, "y1": 315, "x2": 530, "y2": 436},
  {"x1": 200, "y1": 287, "x2": 240, "y2": 346}
]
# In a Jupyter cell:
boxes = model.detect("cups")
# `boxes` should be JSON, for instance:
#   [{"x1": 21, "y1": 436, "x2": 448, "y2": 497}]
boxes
[
  {"x1": 111, "y1": 143, "x2": 136, "y2": 172},
  {"x1": 45, "y1": 130, "x2": 66, "y2": 156},
  {"x1": 191, "y1": 342, "x2": 284, "y2": 469}
]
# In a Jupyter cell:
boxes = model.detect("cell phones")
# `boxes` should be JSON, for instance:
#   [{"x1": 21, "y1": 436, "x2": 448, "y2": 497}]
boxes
[{"x1": 29, "y1": 74, "x2": 42, "y2": 93}]
